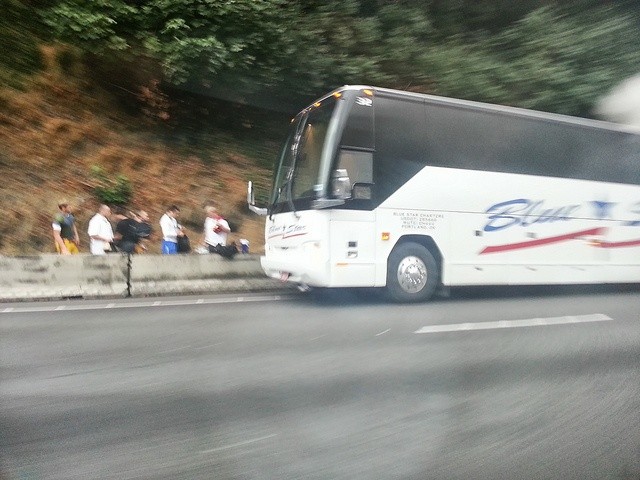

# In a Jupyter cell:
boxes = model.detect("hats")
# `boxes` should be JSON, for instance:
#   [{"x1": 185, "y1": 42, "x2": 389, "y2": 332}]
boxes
[{"x1": 240, "y1": 239, "x2": 249, "y2": 247}]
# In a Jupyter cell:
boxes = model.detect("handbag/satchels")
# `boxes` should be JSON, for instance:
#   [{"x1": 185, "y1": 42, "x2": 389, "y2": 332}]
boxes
[{"x1": 178, "y1": 233, "x2": 192, "y2": 253}]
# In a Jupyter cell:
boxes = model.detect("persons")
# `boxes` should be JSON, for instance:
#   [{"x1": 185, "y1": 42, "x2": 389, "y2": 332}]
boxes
[
  {"x1": 117, "y1": 211, "x2": 150, "y2": 253},
  {"x1": 88, "y1": 204, "x2": 116, "y2": 254},
  {"x1": 53, "y1": 199, "x2": 81, "y2": 254},
  {"x1": 203, "y1": 207, "x2": 231, "y2": 248},
  {"x1": 159, "y1": 205, "x2": 184, "y2": 253}
]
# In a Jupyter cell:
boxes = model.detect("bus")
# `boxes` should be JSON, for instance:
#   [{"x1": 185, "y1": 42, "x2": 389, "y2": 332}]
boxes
[{"x1": 246, "y1": 84, "x2": 639, "y2": 302}]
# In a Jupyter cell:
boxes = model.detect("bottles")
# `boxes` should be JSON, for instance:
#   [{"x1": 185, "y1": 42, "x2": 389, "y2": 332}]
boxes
[{"x1": 239, "y1": 239, "x2": 250, "y2": 254}]
